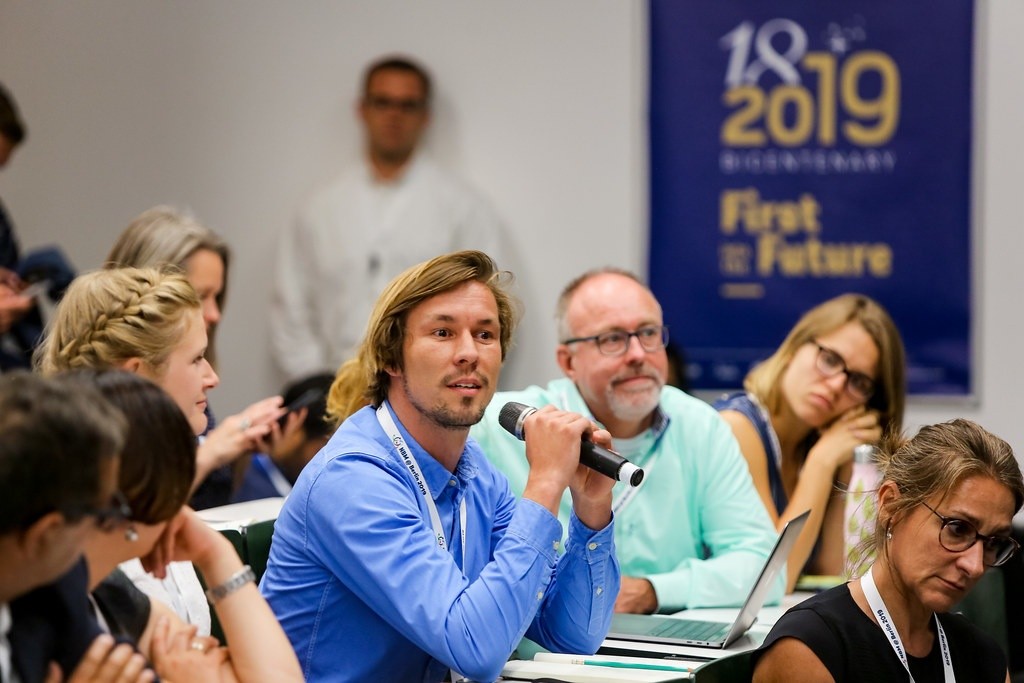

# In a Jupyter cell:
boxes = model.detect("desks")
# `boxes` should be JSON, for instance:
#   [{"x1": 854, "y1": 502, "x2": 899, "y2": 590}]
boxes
[
  {"x1": 501, "y1": 588, "x2": 820, "y2": 683},
  {"x1": 192, "y1": 493, "x2": 289, "y2": 531}
]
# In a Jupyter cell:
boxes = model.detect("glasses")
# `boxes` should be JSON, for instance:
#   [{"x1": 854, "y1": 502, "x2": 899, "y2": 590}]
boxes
[
  {"x1": 369, "y1": 95, "x2": 425, "y2": 110},
  {"x1": 811, "y1": 336, "x2": 874, "y2": 398},
  {"x1": 915, "y1": 499, "x2": 1019, "y2": 566},
  {"x1": 564, "y1": 323, "x2": 671, "y2": 357}
]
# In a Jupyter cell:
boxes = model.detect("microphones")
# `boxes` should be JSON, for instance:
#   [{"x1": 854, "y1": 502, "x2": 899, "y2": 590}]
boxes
[{"x1": 498, "y1": 402, "x2": 644, "y2": 487}]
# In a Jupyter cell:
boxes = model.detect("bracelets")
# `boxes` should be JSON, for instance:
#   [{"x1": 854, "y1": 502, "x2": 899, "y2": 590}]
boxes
[{"x1": 206, "y1": 565, "x2": 255, "y2": 608}]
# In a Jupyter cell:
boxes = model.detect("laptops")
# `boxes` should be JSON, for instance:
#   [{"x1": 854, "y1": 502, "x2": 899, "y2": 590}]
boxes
[{"x1": 605, "y1": 508, "x2": 813, "y2": 650}]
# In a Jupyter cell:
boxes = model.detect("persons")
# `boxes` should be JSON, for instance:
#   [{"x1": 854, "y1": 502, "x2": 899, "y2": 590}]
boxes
[
  {"x1": 713, "y1": 294, "x2": 905, "y2": 596},
  {"x1": 0, "y1": 83, "x2": 53, "y2": 376},
  {"x1": 468, "y1": 270, "x2": 788, "y2": 619},
  {"x1": 267, "y1": 55, "x2": 504, "y2": 475},
  {"x1": 752, "y1": 417, "x2": 1024, "y2": 683},
  {"x1": 256, "y1": 251, "x2": 621, "y2": 683},
  {"x1": 0, "y1": 261, "x2": 309, "y2": 683},
  {"x1": 232, "y1": 371, "x2": 347, "y2": 581},
  {"x1": 108, "y1": 205, "x2": 287, "y2": 648}
]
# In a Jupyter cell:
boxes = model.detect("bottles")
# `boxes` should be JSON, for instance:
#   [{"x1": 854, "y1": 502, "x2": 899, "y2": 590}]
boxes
[{"x1": 842, "y1": 443, "x2": 886, "y2": 580}]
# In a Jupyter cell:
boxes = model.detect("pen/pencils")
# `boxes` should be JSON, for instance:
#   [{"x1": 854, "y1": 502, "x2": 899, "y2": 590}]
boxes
[{"x1": 572, "y1": 659, "x2": 694, "y2": 672}]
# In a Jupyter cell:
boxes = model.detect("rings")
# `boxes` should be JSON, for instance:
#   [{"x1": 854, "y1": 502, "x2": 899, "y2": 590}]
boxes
[
  {"x1": 241, "y1": 418, "x2": 252, "y2": 429},
  {"x1": 191, "y1": 643, "x2": 205, "y2": 651}
]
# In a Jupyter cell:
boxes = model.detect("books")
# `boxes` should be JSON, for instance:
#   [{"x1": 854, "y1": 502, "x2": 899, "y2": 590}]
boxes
[{"x1": 499, "y1": 652, "x2": 716, "y2": 683}]
